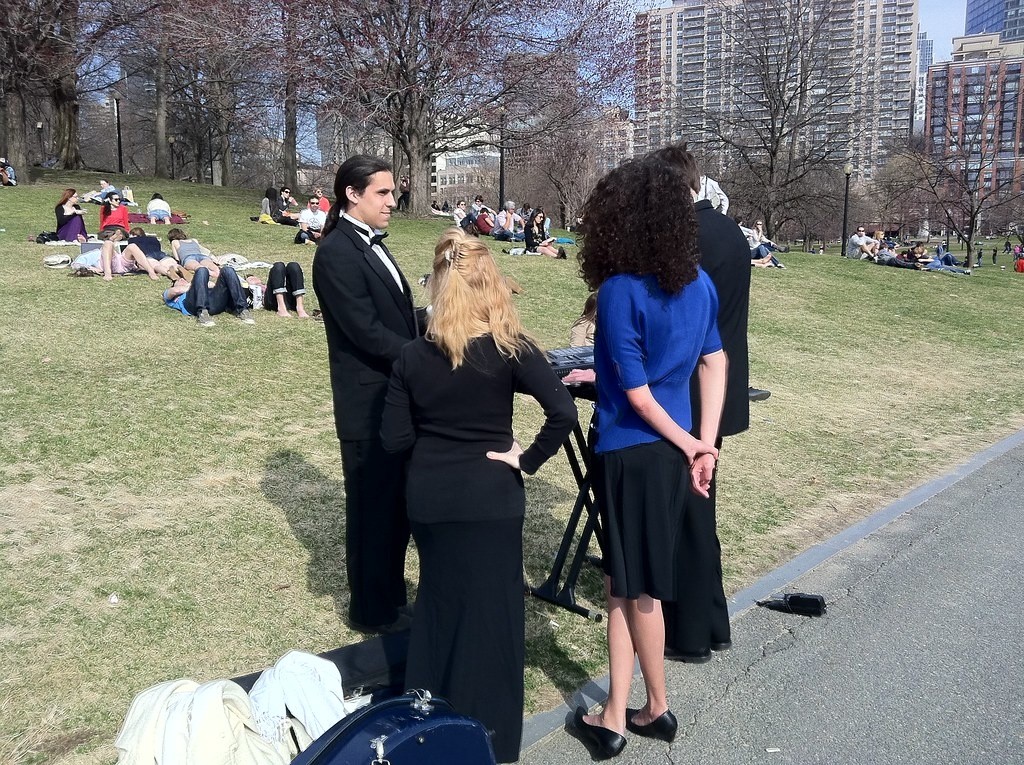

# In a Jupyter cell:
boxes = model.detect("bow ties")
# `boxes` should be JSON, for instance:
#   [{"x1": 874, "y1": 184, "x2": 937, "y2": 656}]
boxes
[{"x1": 343, "y1": 218, "x2": 390, "y2": 247}]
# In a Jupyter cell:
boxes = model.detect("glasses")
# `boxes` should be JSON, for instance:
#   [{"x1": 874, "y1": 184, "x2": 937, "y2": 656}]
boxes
[
  {"x1": 316, "y1": 191, "x2": 323, "y2": 193},
  {"x1": 460, "y1": 204, "x2": 465, "y2": 206},
  {"x1": 857, "y1": 230, "x2": 864, "y2": 232},
  {"x1": 310, "y1": 202, "x2": 319, "y2": 205},
  {"x1": 73, "y1": 196, "x2": 78, "y2": 198},
  {"x1": 536, "y1": 216, "x2": 543, "y2": 219},
  {"x1": 112, "y1": 198, "x2": 120, "y2": 201},
  {"x1": 284, "y1": 191, "x2": 290, "y2": 194},
  {"x1": 756, "y1": 223, "x2": 762, "y2": 225}
]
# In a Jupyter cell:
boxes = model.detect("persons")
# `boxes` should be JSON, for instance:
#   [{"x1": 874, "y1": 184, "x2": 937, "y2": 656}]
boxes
[
  {"x1": 432, "y1": 195, "x2": 566, "y2": 259},
  {"x1": 735, "y1": 213, "x2": 789, "y2": 270},
  {"x1": 0, "y1": 157, "x2": 16, "y2": 186},
  {"x1": 571, "y1": 292, "x2": 598, "y2": 346},
  {"x1": 574, "y1": 160, "x2": 733, "y2": 761},
  {"x1": 564, "y1": 149, "x2": 750, "y2": 661},
  {"x1": 312, "y1": 154, "x2": 431, "y2": 630},
  {"x1": 812, "y1": 245, "x2": 823, "y2": 254},
  {"x1": 379, "y1": 224, "x2": 578, "y2": 764},
  {"x1": 397, "y1": 180, "x2": 409, "y2": 211},
  {"x1": 55, "y1": 178, "x2": 330, "y2": 326},
  {"x1": 846, "y1": 224, "x2": 1024, "y2": 274}
]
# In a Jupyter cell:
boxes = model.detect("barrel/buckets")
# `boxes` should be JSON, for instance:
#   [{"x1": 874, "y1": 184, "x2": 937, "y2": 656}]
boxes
[{"x1": 1000, "y1": 265, "x2": 1005, "y2": 270}]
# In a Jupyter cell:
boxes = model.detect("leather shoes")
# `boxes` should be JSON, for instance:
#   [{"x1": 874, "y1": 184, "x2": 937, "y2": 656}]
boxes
[
  {"x1": 710, "y1": 639, "x2": 731, "y2": 650},
  {"x1": 664, "y1": 641, "x2": 711, "y2": 663}
]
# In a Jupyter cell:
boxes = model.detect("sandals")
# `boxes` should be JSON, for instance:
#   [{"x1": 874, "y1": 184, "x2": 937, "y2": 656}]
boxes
[{"x1": 67, "y1": 267, "x2": 93, "y2": 277}]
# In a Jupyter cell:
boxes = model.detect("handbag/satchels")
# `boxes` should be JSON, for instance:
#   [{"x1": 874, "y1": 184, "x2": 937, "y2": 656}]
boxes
[
  {"x1": 281, "y1": 687, "x2": 496, "y2": 765},
  {"x1": 172, "y1": 210, "x2": 188, "y2": 218}
]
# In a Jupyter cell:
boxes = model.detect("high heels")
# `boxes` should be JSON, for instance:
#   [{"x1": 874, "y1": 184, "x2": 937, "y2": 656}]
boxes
[
  {"x1": 573, "y1": 705, "x2": 628, "y2": 761},
  {"x1": 624, "y1": 707, "x2": 678, "y2": 743}
]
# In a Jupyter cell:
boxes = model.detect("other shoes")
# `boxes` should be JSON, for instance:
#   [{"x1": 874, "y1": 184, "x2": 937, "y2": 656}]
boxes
[
  {"x1": 236, "y1": 309, "x2": 255, "y2": 325},
  {"x1": 561, "y1": 250, "x2": 566, "y2": 259},
  {"x1": 177, "y1": 265, "x2": 193, "y2": 281},
  {"x1": 556, "y1": 246, "x2": 563, "y2": 259},
  {"x1": 197, "y1": 309, "x2": 215, "y2": 326},
  {"x1": 169, "y1": 265, "x2": 179, "y2": 281}
]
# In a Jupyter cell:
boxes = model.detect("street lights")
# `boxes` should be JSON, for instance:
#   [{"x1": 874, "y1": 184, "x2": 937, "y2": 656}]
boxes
[
  {"x1": 841, "y1": 161, "x2": 854, "y2": 256},
  {"x1": 114, "y1": 90, "x2": 123, "y2": 173},
  {"x1": 168, "y1": 135, "x2": 175, "y2": 179},
  {"x1": 36, "y1": 121, "x2": 44, "y2": 163},
  {"x1": 497, "y1": 104, "x2": 506, "y2": 212},
  {"x1": 946, "y1": 209, "x2": 953, "y2": 252}
]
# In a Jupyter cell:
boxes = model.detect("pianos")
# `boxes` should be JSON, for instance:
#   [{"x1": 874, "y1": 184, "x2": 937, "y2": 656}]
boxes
[{"x1": 513, "y1": 341, "x2": 772, "y2": 624}]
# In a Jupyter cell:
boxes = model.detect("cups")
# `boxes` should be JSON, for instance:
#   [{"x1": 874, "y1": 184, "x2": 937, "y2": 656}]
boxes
[{"x1": 288, "y1": 197, "x2": 299, "y2": 207}]
